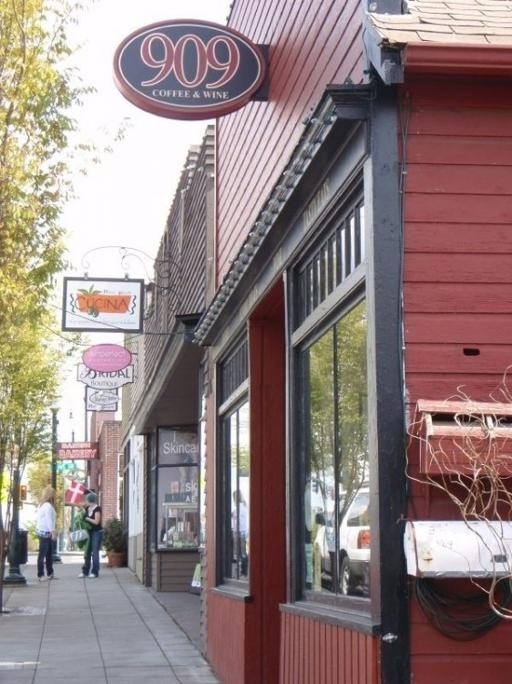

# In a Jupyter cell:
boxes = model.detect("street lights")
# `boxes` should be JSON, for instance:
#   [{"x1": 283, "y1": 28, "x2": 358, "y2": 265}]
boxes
[
  {"x1": 67, "y1": 409, "x2": 77, "y2": 550},
  {"x1": 48, "y1": 390, "x2": 64, "y2": 563}
]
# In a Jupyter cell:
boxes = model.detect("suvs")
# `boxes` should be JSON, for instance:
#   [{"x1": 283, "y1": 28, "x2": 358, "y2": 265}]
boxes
[{"x1": 313, "y1": 488, "x2": 372, "y2": 597}]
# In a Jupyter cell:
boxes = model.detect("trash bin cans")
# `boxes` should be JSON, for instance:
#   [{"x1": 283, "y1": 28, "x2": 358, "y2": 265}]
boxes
[{"x1": 8, "y1": 531, "x2": 28, "y2": 565}]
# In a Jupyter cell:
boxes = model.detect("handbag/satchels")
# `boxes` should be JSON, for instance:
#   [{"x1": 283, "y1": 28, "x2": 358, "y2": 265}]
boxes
[{"x1": 70, "y1": 514, "x2": 89, "y2": 542}]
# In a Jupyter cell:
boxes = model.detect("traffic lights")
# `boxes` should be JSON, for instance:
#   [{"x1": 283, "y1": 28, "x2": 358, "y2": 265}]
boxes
[{"x1": 21, "y1": 485, "x2": 27, "y2": 500}]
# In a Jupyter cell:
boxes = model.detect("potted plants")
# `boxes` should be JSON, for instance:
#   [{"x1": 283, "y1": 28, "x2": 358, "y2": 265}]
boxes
[{"x1": 101, "y1": 518, "x2": 128, "y2": 566}]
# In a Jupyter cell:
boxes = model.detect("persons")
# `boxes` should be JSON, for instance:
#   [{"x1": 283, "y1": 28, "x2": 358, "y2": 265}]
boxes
[
  {"x1": 231, "y1": 490, "x2": 247, "y2": 554},
  {"x1": 77, "y1": 493, "x2": 101, "y2": 578},
  {"x1": 35, "y1": 486, "x2": 58, "y2": 581}
]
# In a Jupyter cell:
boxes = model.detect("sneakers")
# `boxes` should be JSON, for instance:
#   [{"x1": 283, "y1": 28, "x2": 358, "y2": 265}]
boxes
[
  {"x1": 78, "y1": 572, "x2": 84, "y2": 578},
  {"x1": 39, "y1": 576, "x2": 48, "y2": 582},
  {"x1": 88, "y1": 573, "x2": 95, "y2": 578}
]
